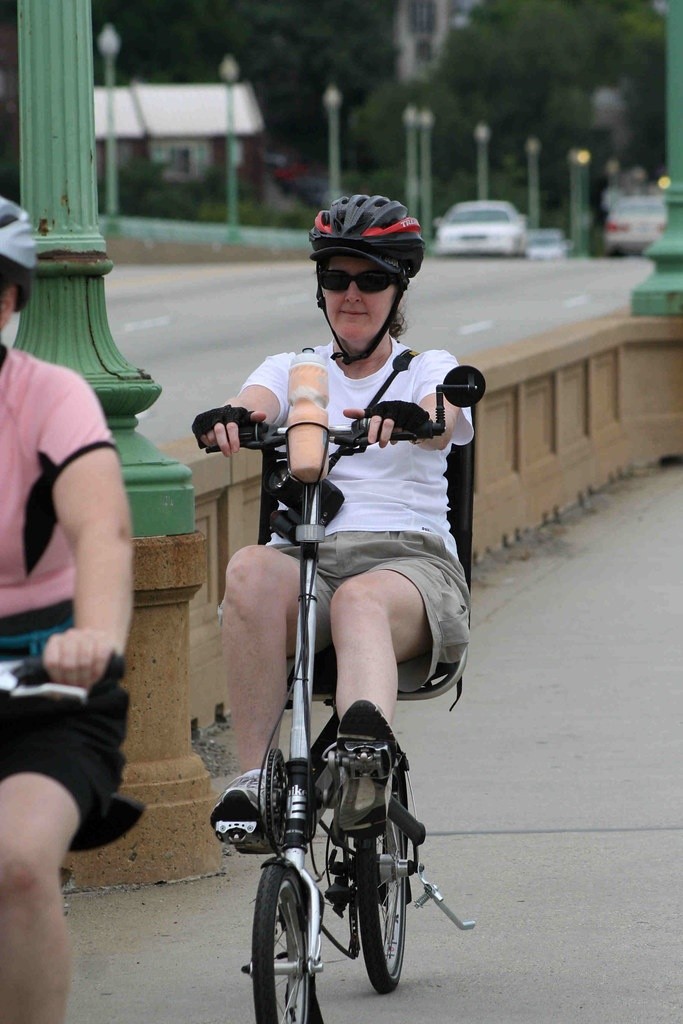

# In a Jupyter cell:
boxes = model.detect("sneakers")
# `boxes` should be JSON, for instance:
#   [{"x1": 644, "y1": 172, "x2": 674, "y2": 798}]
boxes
[
  {"x1": 210, "y1": 773, "x2": 284, "y2": 854},
  {"x1": 337, "y1": 700, "x2": 397, "y2": 840}
]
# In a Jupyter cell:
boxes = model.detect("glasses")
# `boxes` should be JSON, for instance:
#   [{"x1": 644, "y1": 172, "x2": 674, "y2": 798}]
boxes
[{"x1": 320, "y1": 270, "x2": 398, "y2": 292}]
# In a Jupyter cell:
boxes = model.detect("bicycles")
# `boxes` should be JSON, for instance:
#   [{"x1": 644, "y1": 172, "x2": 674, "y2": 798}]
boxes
[{"x1": 200, "y1": 365, "x2": 487, "y2": 1023}]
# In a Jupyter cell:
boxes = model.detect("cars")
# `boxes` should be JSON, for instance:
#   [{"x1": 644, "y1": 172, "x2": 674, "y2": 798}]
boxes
[
  {"x1": 523, "y1": 227, "x2": 570, "y2": 263},
  {"x1": 430, "y1": 198, "x2": 527, "y2": 260},
  {"x1": 601, "y1": 194, "x2": 670, "y2": 257}
]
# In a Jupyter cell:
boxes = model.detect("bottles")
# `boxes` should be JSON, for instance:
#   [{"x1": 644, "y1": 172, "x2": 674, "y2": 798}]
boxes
[{"x1": 287, "y1": 348, "x2": 329, "y2": 484}]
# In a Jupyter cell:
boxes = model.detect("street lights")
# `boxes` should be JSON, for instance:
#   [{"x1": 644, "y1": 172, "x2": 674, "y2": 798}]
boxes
[
  {"x1": 526, "y1": 133, "x2": 541, "y2": 230},
  {"x1": 221, "y1": 51, "x2": 241, "y2": 247},
  {"x1": 326, "y1": 85, "x2": 341, "y2": 209},
  {"x1": 417, "y1": 105, "x2": 434, "y2": 244},
  {"x1": 475, "y1": 120, "x2": 490, "y2": 200},
  {"x1": 404, "y1": 103, "x2": 418, "y2": 217},
  {"x1": 100, "y1": 23, "x2": 120, "y2": 238}
]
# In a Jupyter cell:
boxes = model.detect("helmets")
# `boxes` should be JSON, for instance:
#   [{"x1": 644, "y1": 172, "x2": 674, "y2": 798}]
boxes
[
  {"x1": 309, "y1": 195, "x2": 425, "y2": 278},
  {"x1": 0, "y1": 195, "x2": 37, "y2": 312}
]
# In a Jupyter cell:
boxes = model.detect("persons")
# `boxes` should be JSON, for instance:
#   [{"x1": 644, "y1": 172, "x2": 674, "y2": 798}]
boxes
[
  {"x1": 192, "y1": 194, "x2": 474, "y2": 853},
  {"x1": 0, "y1": 197, "x2": 133, "y2": 1024}
]
